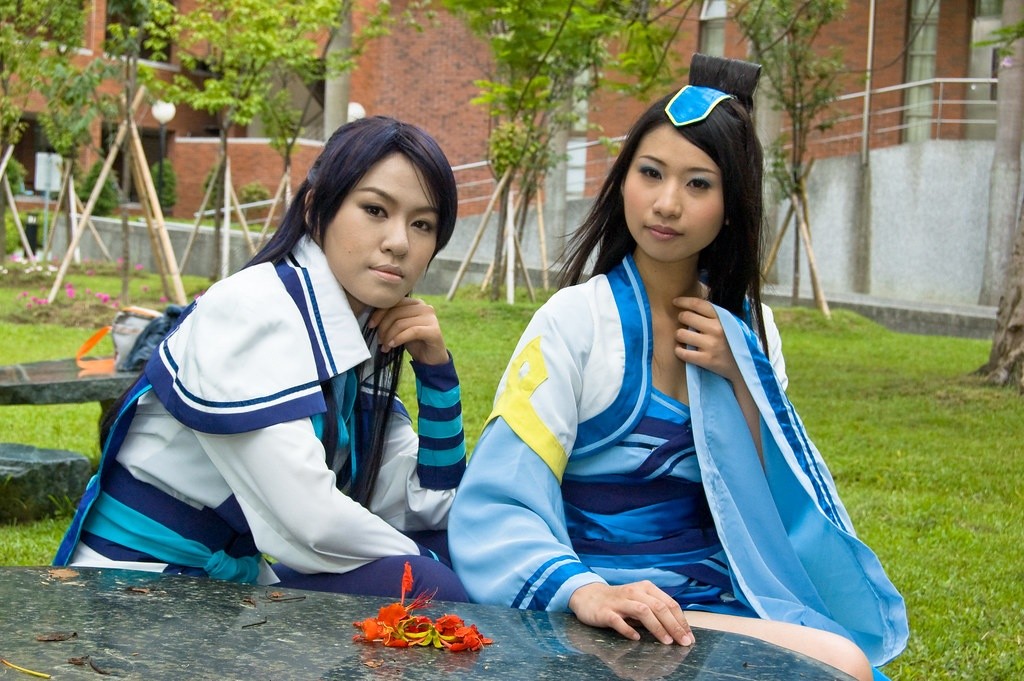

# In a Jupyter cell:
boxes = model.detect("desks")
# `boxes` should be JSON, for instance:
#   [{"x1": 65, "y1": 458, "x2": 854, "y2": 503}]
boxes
[{"x1": 0, "y1": 564, "x2": 859, "y2": 681}]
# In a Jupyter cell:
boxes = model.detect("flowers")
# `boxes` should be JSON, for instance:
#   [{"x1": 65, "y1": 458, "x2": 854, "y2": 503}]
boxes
[{"x1": 352, "y1": 561, "x2": 494, "y2": 652}]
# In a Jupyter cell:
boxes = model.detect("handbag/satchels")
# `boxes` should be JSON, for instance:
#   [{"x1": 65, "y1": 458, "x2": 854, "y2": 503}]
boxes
[
  {"x1": 123, "y1": 304, "x2": 190, "y2": 376},
  {"x1": 74, "y1": 306, "x2": 163, "y2": 374}
]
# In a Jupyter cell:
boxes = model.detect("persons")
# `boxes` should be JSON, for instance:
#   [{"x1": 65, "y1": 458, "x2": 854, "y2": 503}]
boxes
[
  {"x1": 48, "y1": 113, "x2": 474, "y2": 605},
  {"x1": 448, "y1": 53, "x2": 910, "y2": 681}
]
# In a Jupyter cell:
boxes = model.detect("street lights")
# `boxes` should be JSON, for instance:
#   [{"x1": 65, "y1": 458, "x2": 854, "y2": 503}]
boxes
[{"x1": 151, "y1": 98, "x2": 177, "y2": 206}]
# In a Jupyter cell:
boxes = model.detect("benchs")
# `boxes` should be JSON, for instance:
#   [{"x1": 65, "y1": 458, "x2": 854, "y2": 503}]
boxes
[{"x1": 0, "y1": 354, "x2": 141, "y2": 452}]
